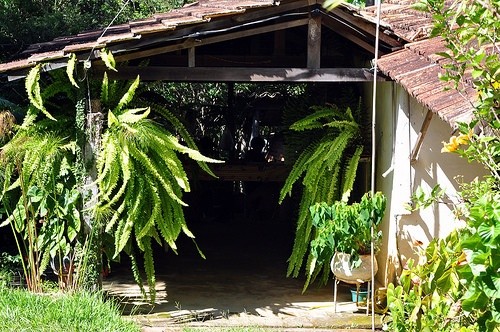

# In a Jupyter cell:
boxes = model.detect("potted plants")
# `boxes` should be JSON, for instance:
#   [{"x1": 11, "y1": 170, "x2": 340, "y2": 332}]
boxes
[
  {"x1": 352, "y1": 287, "x2": 371, "y2": 304},
  {"x1": 315, "y1": 191, "x2": 387, "y2": 313}
]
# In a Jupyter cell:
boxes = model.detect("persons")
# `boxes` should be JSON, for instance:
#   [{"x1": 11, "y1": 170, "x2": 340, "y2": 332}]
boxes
[
  {"x1": 100, "y1": 245, "x2": 108, "y2": 280},
  {"x1": 243, "y1": 137, "x2": 267, "y2": 162}
]
